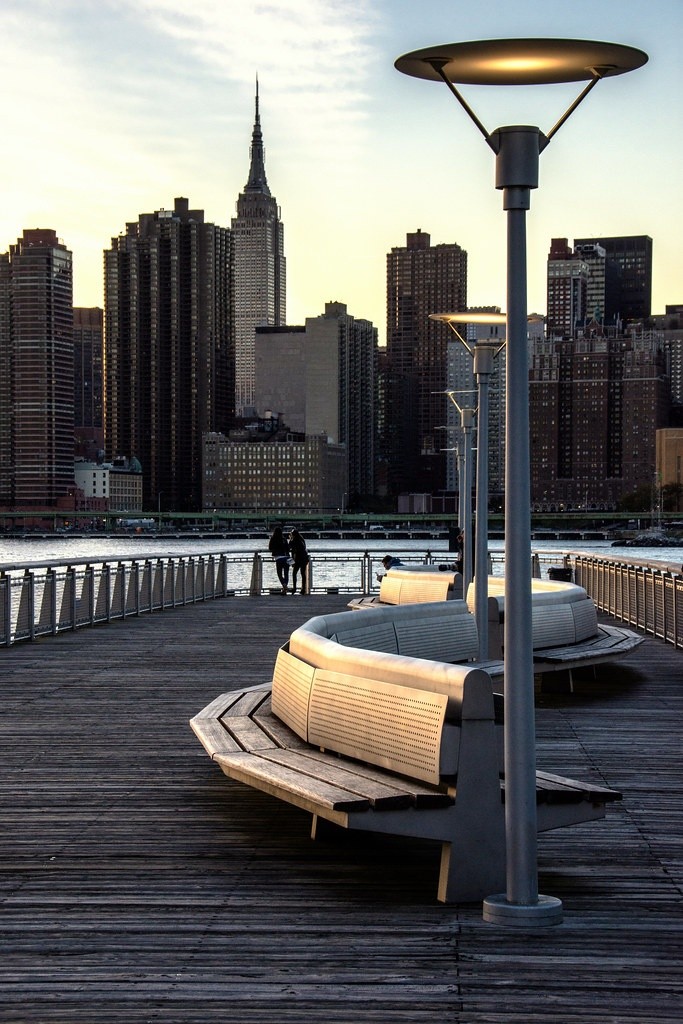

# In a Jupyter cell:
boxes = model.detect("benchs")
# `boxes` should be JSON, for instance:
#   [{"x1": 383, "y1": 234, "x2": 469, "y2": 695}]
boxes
[{"x1": 191, "y1": 562, "x2": 643, "y2": 901}]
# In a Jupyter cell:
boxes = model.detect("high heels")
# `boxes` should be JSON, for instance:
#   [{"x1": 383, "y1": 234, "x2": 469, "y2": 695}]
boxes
[
  {"x1": 300, "y1": 589, "x2": 305, "y2": 594},
  {"x1": 289, "y1": 588, "x2": 296, "y2": 593}
]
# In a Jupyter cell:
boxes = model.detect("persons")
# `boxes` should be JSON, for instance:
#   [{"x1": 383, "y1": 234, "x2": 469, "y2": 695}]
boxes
[
  {"x1": 288, "y1": 528, "x2": 309, "y2": 595},
  {"x1": 377, "y1": 555, "x2": 406, "y2": 582},
  {"x1": 269, "y1": 527, "x2": 290, "y2": 596}
]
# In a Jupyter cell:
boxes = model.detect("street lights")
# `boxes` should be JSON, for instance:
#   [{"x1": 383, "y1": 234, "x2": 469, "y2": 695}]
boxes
[
  {"x1": 391, "y1": 28, "x2": 650, "y2": 934},
  {"x1": 432, "y1": 386, "x2": 503, "y2": 599},
  {"x1": 428, "y1": 309, "x2": 546, "y2": 662}
]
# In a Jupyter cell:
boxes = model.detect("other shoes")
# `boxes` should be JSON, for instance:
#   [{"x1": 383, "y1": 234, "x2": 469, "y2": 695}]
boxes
[{"x1": 281, "y1": 586, "x2": 287, "y2": 595}]
